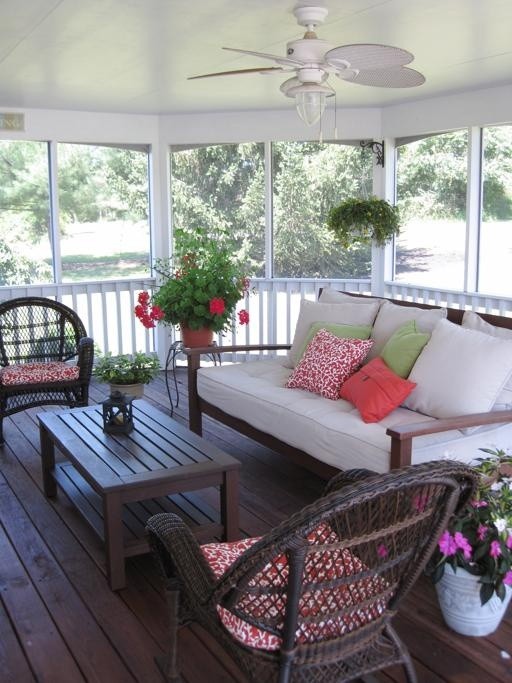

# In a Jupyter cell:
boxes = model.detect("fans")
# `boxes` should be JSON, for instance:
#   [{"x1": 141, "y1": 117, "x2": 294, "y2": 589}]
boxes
[{"x1": 186, "y1": 5, "x2": 425, "y2": 97}]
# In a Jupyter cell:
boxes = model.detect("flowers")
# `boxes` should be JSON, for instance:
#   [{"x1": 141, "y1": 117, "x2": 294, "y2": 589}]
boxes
[
  {"x1": 134, "y1": 225, "x2": 251, "y2": 333},
  {"x1": 378, "y1": 446, "x2": 512, "y2": 602}
]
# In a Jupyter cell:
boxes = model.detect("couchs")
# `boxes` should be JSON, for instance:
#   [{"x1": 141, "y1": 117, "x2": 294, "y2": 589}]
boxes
[{"x1": 179, "y1": 287, "x2": 512, "y2": 487}]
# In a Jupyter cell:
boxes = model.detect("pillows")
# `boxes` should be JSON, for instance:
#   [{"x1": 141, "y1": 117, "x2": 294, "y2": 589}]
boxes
[{"x1": 279, "y1": 287, "x2": 512, "y2": 423}]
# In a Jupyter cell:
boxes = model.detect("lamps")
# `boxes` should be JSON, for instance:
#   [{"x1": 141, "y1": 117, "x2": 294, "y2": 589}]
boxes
[{"x1": 285, "y1": 69, "x2": 337, "y2": 126}]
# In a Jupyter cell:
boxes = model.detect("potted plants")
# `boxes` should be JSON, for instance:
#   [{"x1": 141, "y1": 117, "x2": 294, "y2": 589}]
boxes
[
  {"x1": 92, "y1": 346, "x2": 161, "y2": 397},
  {"x1": 327, "y1": 197, "x2": 405, "y2": 254}
]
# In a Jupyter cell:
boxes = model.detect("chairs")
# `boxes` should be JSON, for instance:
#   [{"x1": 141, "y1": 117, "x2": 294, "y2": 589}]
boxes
[
  {"x1": 144, "y1": 457, "x2": 478, "y2": 682},
  {"x1": 0, "y1": 295, "x2": 94, "y2": 444}
]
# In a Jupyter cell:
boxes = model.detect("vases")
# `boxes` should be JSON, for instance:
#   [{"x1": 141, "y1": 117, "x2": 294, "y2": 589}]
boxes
[
  {"x1": 179, "y1": 318, "x2": 211, "y2": 347},
  {"x1": 432, "y1": 561, "x2": 512, "y2": 637}
]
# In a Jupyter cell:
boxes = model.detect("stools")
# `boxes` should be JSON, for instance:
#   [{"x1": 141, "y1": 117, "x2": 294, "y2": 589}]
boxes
[{"x1": 165, "y1": 341, "x2": 223, "y2": 417}]
